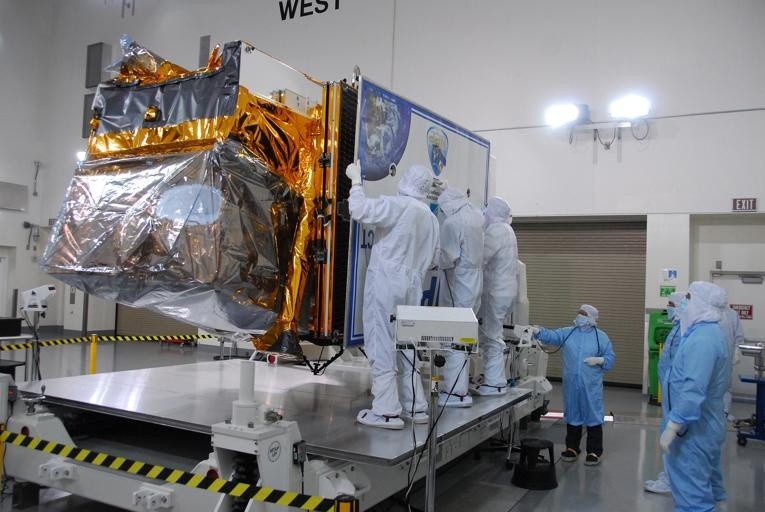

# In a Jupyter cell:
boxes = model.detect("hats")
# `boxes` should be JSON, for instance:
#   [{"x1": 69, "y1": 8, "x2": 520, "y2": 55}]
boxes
[
  {"x1": 578, "y1": 303, "x2": 599, "y2": 322},
  {"x1": 667, "y1": 280, "x2": 729, "y2": 322}
]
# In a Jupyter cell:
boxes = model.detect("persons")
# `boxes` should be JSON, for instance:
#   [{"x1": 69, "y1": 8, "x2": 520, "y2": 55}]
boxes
[
  {"x1": 718, "y1": 306, "x2": 744, "y2": 422},
  {"x1": 345, "y1": 159, "x2": 442, "y2": 429},
  {"x1": 532, "y1": 304, "x2": 615, "y2": 466},
  {"x1": 437, "y1": 188, "x2": 486, "y2": 407},
  {"x1": 645, "y1": 292, "x2": 686, "y2": 493},
  {"x1": 659, "y1": 281, "x2": 731, "y2": 512},
  {"x1": 477, "y1": 196, "x2": 518, "y2": 396}
]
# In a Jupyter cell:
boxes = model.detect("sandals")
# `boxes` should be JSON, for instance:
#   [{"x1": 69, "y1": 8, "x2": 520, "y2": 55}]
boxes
[
  {"x1": 560, "y1": 447, "x2": 576, "y2": 462},
  {"x1": 585, "y1": 453, "x2": 599, "y2": 466}
]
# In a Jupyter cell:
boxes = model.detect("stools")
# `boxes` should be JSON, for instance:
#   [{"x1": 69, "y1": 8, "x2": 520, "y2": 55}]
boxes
[{"x1": 511, "y1": 438, "x2": 558, "y2": 490}]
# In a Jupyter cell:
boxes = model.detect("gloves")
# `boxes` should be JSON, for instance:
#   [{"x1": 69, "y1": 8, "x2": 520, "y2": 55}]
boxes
[
  {"x1": 583, "y1": 355, "x2": 604, "y2": 366},
  {"x1": 524, "y1": 324, "x2": 541, "y2": 337},
  {"x1": 345, "y1": 159, "x2": 362, "y2": 184},
  {"x1": 660, "y1": 421, "x2": 678, "y2": 453}
]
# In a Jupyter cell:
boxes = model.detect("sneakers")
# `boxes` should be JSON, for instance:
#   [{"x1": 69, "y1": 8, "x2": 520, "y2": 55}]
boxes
[
  {"x1": 407, "y1": 408, "x2": 429, "y2": 426},
  {"x1": 473, "y1": 383, "x2": 508, "y2": 397},
  {"x1": 436, "y1": 391, "x2": 472, "y2": 408},
  {"x1": 356, "y1": 409, "x2": 405, "y2": 432},
  {"x1": 643, "y1": 475, "x2": 673, "y2": 496}
]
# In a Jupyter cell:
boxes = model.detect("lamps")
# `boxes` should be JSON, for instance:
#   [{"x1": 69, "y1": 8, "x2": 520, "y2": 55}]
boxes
[{"x1": 545, "y1": 92, "x2": 649, "y2": 150}]
